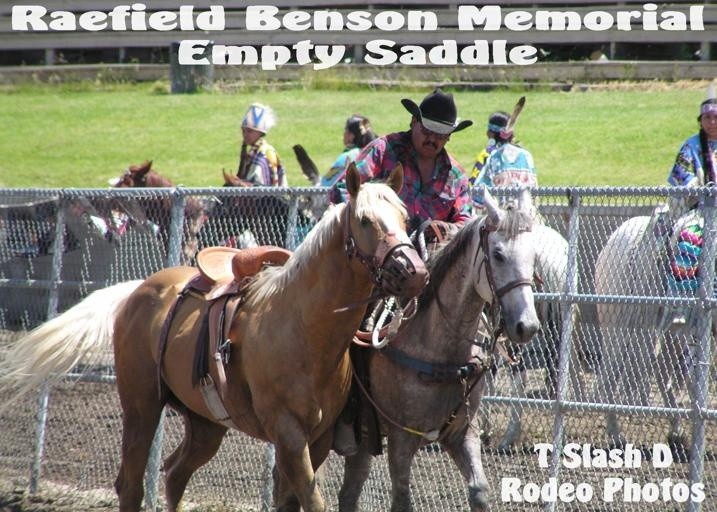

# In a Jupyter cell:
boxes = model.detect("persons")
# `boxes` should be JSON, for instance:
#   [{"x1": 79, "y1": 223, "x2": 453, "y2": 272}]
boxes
[
  {"x1": 469, "y1": 111, "x2": 536, "y2": 215},
  {"x1": 217, "y1": 102, "x2": 290, "y2": 219},
  {"x1": 319, "y1": 112, "x2": 377, "y2": 190},
  {"x1": 655, "y1": 97, "x2": 716, "y2": 325},
  {"x1": 329, "y1": 88, "x2": 471, "y2": 428}
]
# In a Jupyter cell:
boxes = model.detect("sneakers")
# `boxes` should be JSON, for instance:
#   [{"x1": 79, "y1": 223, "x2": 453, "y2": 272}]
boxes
[{"x1": 332, "y1": 413, "x2": 360, "y2": 458}]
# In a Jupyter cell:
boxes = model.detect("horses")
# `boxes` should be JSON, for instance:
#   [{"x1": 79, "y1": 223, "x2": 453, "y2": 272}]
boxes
[
  {"x1": 0, "y1": 160, "x2": 432, "y2": 512},
  {"x1": 325, "y1": 186, "x2": 546, "y2": 512},
  {"x1": 89, "y1": 158, "x2": 208, "y2": 248},
  {"x1": 195, "y1": 166, "x2": 313, "y2": 252},
  {"x1": 483, "y1": 213, "x2": 581, "y2": 455},
  {"x1": 591, "y1": 213, "x2": 717, "y2": 456}
]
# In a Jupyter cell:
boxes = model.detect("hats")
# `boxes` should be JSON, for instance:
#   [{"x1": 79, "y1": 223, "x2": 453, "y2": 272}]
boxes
[
  {"x1": 240, "y1": 102, "x2": 269, "y2": 137},
  {"x1": 401, "y1": 87, "x2": 473, "y2": 135}
]
